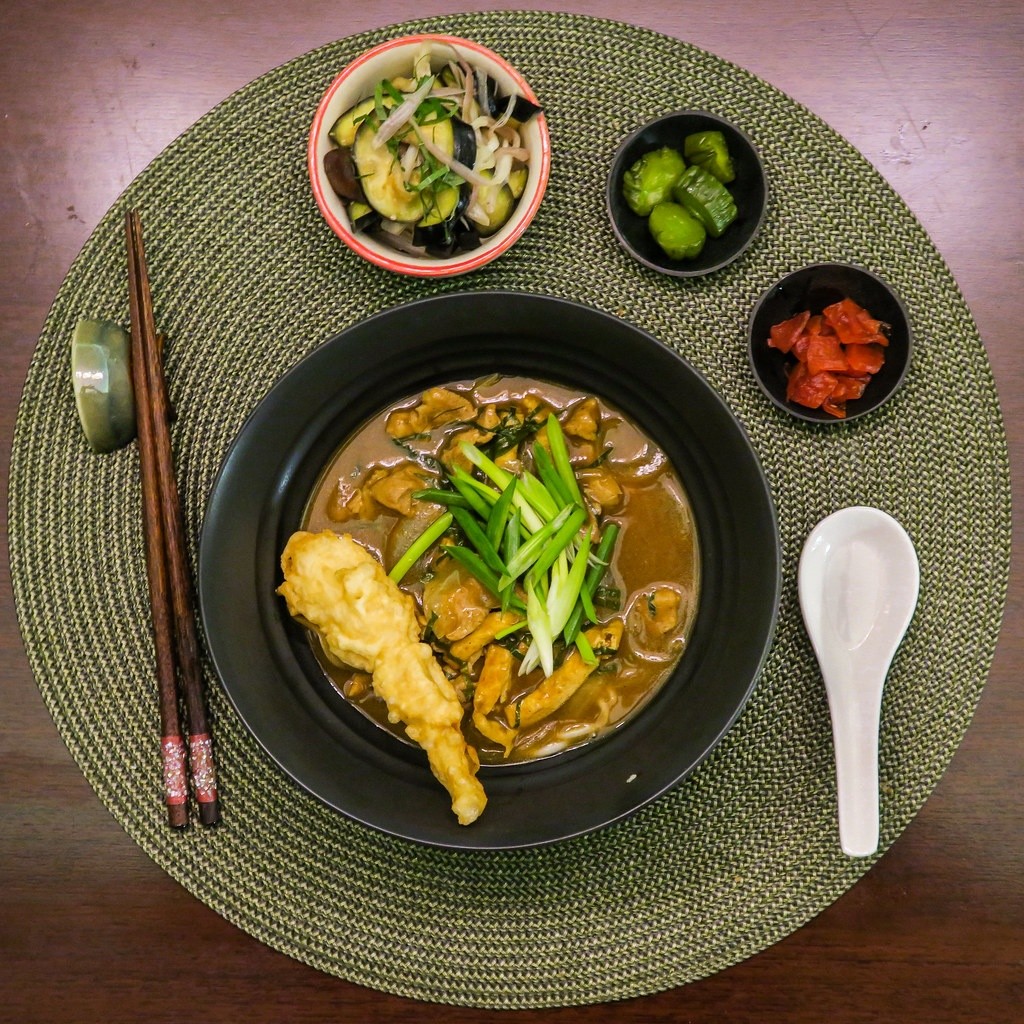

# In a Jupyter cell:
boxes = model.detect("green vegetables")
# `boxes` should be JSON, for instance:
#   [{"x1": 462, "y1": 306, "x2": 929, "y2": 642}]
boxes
[{"x1": 386, "y1": 414, "x2": 621, "y2": 679}]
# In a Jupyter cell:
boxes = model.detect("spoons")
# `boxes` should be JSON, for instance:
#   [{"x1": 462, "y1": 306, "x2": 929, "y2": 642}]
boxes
[{"x1": 797, "y1": 506, "x2": 920, "y2": 858}]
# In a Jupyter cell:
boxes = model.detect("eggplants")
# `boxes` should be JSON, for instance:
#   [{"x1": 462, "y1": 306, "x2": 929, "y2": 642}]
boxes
[{"x1": 323, "y1": 62, "x2": 545, "y2": 262}]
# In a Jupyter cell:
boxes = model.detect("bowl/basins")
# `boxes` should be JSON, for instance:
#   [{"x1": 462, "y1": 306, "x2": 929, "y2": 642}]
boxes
[
  {"x1": 69, "y1": 316, "x2": 136, "y2": 460},
  {"x1": 605, "y1": 110, "x2": 768, "y2": 278},
  {"x1": 746, "y1": 262, "x2": 912, "y2": 424},
  {"x1": 196, "y1": 288, "x2": 782, "y2": 852},
  {"x1": 307, "y1": 35, "x2": 551, "y2": 281}
]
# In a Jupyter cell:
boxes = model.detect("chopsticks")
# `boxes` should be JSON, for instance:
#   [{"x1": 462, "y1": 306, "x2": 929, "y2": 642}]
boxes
[{"x1": 123, "y1": 210, "x2": 222, "y2": 832}]
